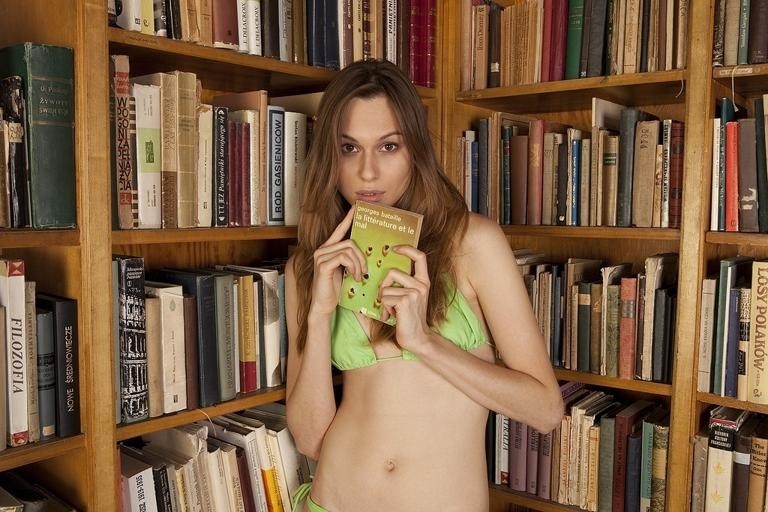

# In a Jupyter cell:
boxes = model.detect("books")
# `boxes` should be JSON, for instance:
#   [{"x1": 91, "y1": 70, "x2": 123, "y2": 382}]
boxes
[
  {"x1": 111, "y1": 245, "x2": 303, "y2": 424},
  {"x1": 337, "y1": 200, "x2": 425, "y2": 326},
  {"x1": 485, "y1": 380, "x2": 669, "y2": 512},
  {"x1": 109, "y1": 56, "x2": 317, "y2": 229},
  {"x1": 0, "y1": 258, "x2": 80, "y2": 453},
  {"x1": 696, "y1": 256, "x2": 768, "y2": 406},
  {"x1": 712, "y1": 0, "x2": 768, "y2": 67},
  {"x1": 710, "y1": 93, "x2": 768, "y2": 234},
  {"x1": 117, "y1": 402, "x2": 318, "y2": 512},
  {"x1": 459, "y1": 0, "x2": 691, "y2": 91},
  {"x1": 692, "y1": 405, "x2": 768, "y2": 512},
  {"x1": 511, "y1": 248, "x2": 679, "y2": 386},
  {"x1": 0, "y1": 471, "x2": 77, "y2": 512},
  {"x1": 0, "y1": 42, "x2": 75, "y2": 230},
  {"x1": 452, "y1": 97, "x2": 685, "y2": 228}
]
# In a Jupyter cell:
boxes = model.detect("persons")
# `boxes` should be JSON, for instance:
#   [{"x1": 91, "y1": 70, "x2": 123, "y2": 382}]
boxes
[{"x1": 285, "y1": 59, "x2": 564, "y2": 512}]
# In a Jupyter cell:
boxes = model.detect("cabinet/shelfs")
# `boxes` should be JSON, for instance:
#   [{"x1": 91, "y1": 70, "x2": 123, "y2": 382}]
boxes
[{"x1": 0, "y1": 1, "x2": 768, "y2": 512}]
